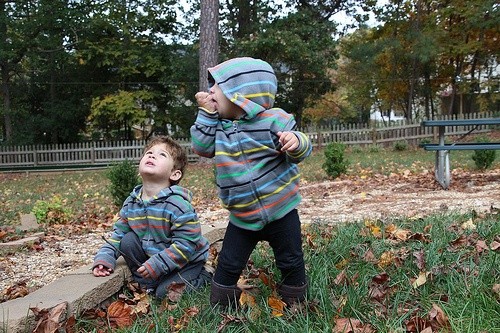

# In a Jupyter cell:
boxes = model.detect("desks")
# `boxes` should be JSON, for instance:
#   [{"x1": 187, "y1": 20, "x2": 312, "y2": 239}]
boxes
[{"x1": 417, "y1": 118, "x2": 500, "y2": 189}]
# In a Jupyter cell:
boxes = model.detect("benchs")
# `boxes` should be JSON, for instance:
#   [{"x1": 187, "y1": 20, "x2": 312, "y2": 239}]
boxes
[{"x1": 419, "y1": 142, "x2": 500, "y2": 151}]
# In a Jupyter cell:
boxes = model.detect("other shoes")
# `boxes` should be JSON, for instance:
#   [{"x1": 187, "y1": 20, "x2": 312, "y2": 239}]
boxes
[
  {"x1": 146, "y1": 288, "x2": 155, "y2": 294},
  {"x1": 203, "y1": 263, "x2": 216, "y2": 276}
]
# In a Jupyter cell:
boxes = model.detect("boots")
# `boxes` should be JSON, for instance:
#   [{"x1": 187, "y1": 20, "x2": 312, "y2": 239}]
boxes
[
  {"x1": 280, "y1": 282, "x2": 309, "y2": 308},
  {"x1": 210, "y1": 278, "x2": 243, "y2": 311}
]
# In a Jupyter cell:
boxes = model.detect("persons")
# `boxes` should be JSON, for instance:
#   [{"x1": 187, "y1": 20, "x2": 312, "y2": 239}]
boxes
[
  {"x1": 91, "y1": 135, "x2": 215, "y2": 303},
  {"x1": 190, "y1": 57, "x2": 313, "y2": 310}
]
